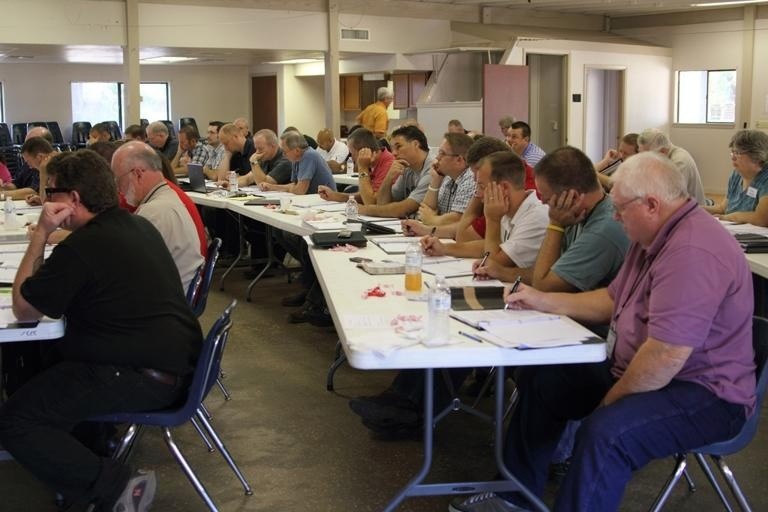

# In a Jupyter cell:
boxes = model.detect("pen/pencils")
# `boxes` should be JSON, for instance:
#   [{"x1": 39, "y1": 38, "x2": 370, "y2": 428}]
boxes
[
  {"x1": 458, "y1": 331, "x2": 482, "y2": 342},
  {"x1": 503, "y1": 276, "x2": 521, "y2": 311},
  {"x1": 406, "y1": 215, "x2": 410, "y2": 233},
  {"x1": 319, "y1": 182, "x2": 330, "y2": 200},
  {"x1": 425, "y1": 227, "x2": 436, "y2": 252},
  {"x1": 471, "y1": 252, "x2": 489, "y2": 281}
]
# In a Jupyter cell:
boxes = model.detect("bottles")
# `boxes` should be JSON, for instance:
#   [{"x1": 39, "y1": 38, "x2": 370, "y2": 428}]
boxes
[
  {"x1": 346, "y1": 157, "x2": 354, "y2": 176},
  {"x1": 4, "y1": 195, "x2": 16, "y2": 229},
  {"x1": 426, "y1": 275, "x2": 452, "y2": 344},
  {"x1": 403, "y1": 237, "x2": 424, "y2": 292},
  {"x1": 227, "y1": 170, "x2": 238, "y2": 193},
  {"x1": 344, "y1": 196, "x2": 359, "y2": 220}
]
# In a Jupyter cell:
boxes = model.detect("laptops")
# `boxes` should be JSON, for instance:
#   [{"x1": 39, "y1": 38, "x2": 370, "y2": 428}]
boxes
[
  {"x1": 310, "y1": 231, "x2": 368, "y2": 247},
  {"x1": 187, "y1": 163, "x2": 227, "y2": 194}
]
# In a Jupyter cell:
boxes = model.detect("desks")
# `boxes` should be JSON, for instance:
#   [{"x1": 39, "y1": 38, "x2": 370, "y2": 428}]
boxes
[
  {"x1": 181, "y1": 174, "x2": 608, "y2": 510},
  {"x1": 712, "y1": 214, "x2": 768, "y2": 327},
  {"x1": 1, "y1": 198, "x2": 64, "y2": 343}
]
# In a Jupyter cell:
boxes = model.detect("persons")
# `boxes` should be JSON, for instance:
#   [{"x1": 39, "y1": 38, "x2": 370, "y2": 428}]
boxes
[
  {"x1": 348, "y1": 125, "x2": 540, "y2": 243},
  {"x1": 450, "y1": 151, "x2": 755, "y2": 511},
  {"x1": 350, "y1": 153, "x2": 551, "y2": 440},
  {"x1": 1, "y1": 150, "x2": 203, "y2": 509},
  {"x1": 699, "y1": 130, "x2": 767, "y2": 227},
  {"x1": 594, "y1": 129, "x2": 706, "y2": 206},
  {"x1": 0, "y1": 121, "x2": 208, "y2": 302},
  {"x1": 506, "y1": 121, "x2": 546, "y2": 168},
  {"x1": 448, "y1": 120, "x2": 472, "y2": 136},
  {"x1": 498, "y1": 115, "x2": 515, "y2": 147},
  {"x1": 202, "y1": 116, "x2": 349, "y2": 282},
  {"x1": 473, "y1": 147, "x2": 631, "y2": 472}
]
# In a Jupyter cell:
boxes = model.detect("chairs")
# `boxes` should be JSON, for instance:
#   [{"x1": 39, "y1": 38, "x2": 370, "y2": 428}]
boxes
[
  {"x1": 651, "y1": 366, "x2": 768, "y2": 511},
  {"x1": 0, "y1": 119, "x2": 201, "y2": 143},
  {"x1": 61, "y1": 222, "x2": 251, "y2": 511}
]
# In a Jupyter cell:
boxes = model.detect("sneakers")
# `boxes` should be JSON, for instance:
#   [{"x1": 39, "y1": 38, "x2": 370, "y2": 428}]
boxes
[
  {"x1": 348, "y1": 394, "x2": 425, "y2": 437},
  {"x1": 282, "y1": 291, "x2": 334, "y2": 328},
  {"x1": 449, "y1": 492, "x2": 524, "y2": 511},
  {"x1": 115, "y1": 467, "x2": 158, "y2": 510}
]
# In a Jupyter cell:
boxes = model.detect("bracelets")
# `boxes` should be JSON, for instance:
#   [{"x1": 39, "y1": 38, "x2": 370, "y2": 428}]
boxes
[{"x1": 545, "y1": 224, "x2": 564, "y2": 232}]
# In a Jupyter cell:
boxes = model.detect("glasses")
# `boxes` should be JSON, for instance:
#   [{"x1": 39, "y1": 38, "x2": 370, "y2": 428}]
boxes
[{"x1": 44, "y1": 185, "x2": 70, "y2": 198}]
